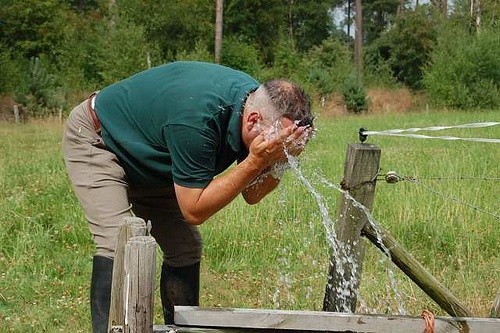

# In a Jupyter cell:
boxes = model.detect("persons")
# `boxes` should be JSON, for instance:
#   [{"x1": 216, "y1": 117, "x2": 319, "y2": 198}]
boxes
[{"x1": 63, "y1": 60, "x2": 316, "y2": 332}]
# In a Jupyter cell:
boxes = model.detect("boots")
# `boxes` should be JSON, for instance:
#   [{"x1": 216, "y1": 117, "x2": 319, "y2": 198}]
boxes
[
  {"x1": 89, "y1": 254, "x2": 113, "y2": 333},
  {"x1": 159, "y1": 260, "x2": 200, "y2": 326}
]
